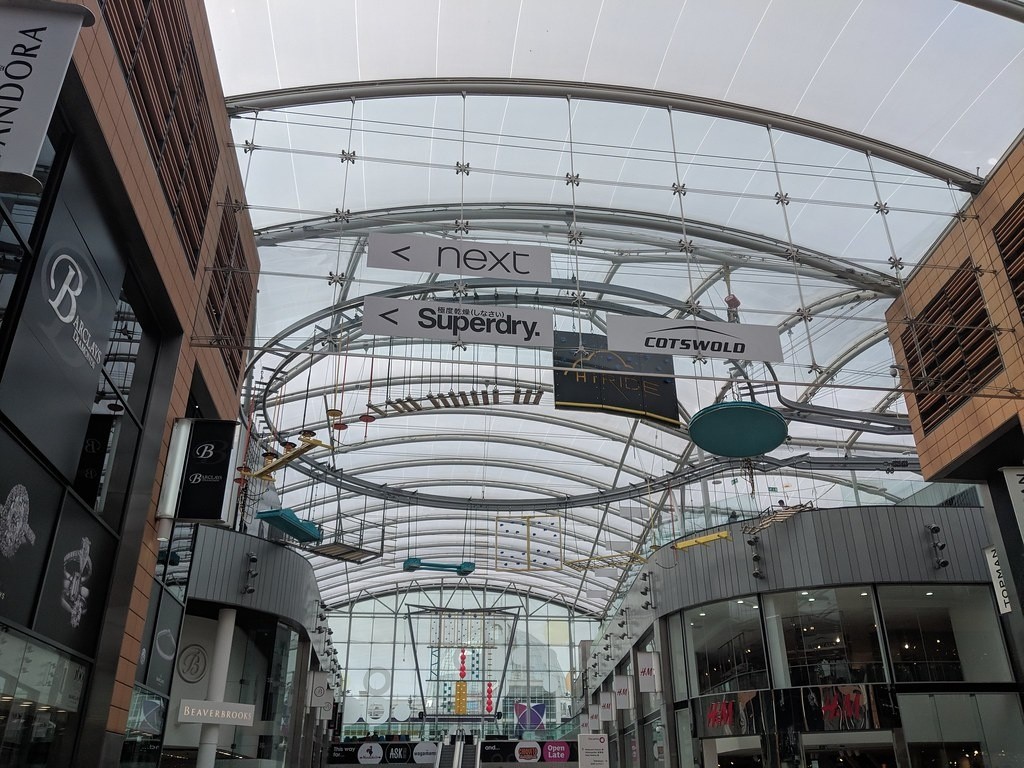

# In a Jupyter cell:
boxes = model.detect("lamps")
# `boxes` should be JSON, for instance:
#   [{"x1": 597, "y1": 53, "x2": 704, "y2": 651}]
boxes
[
  {"x1": 640, "y1": 571, "x2": 653, "y2": 610},
  {"x1": 315, "y1": 599, "x2": 342, "y2": 678},
  {"x1": 656, "y1": 725, "x2": 664, "y2": 733},
  {"x1": 747, "y1": 536, "x2": 762, "y2": 578},
  {"x1": 926, "y1": 524, "x2": 949, "y2": 569},
  {"x1": 241, "y1": 552, "x2": 258, "y2": 594},
  {"x1": 582, "y1": 607, "x2": 631, "y2": 689}
]
